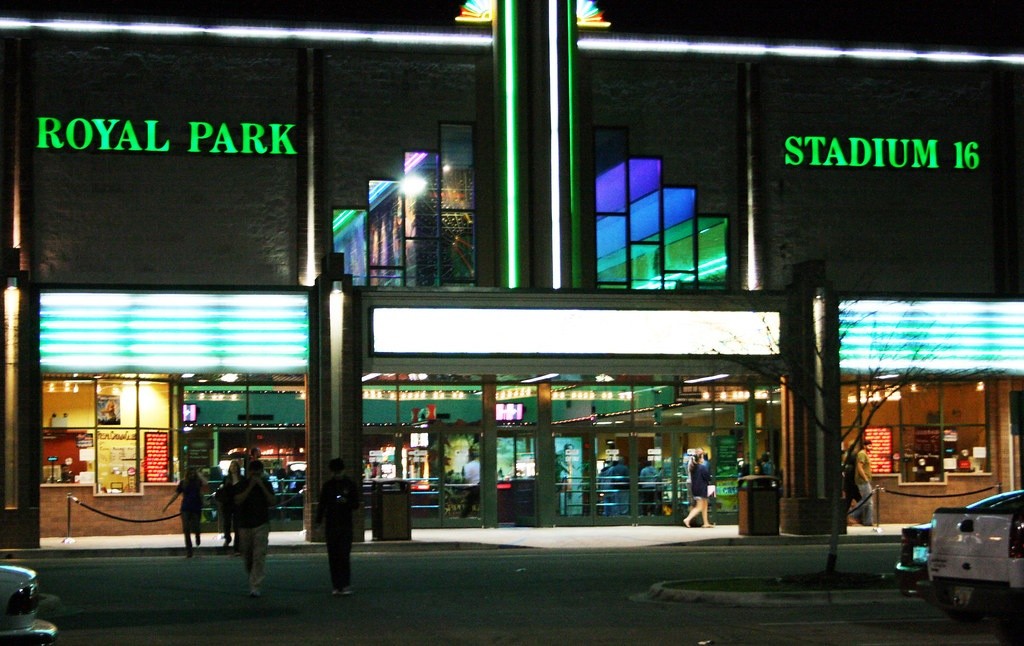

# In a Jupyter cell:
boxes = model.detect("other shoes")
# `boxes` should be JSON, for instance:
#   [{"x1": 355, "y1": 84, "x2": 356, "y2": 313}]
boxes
[
  {"x1": 250, "y1": 586, "x2": 261, "y2": 597},
  {"x1": 196, "y1": 535, "x2": 200, "y2": 545},
  {"x1": 333, "y1": 588, "x2": 354, "y2": 595},
  {"x1": 187, "y1": 549, "x2": 193, "y2": 557},
  {"x1": 224, "y1": 538, "x2": 231, "y2": 547}
]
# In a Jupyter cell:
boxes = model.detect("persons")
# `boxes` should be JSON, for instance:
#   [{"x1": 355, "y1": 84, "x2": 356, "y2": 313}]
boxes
[
  {"x1": 230, "y1": 461, "x2": 278, "y2": 598},
  {"x1": 849, "y1": 440, "x2": 876, "y2": 526},
  {"x1": 162, "y1": 465, "x2": 209, "y2": 558},
  {"x1": 61, "y1": 463, "x2": 72, "y2": 482},
  {"x1": 249, "y1": 445, "x2": 261, "y2": 461},
  {"x1": 316, "y1": 458, "x2": 359, "y2": 595},
  {"x1": 755, "y1": 450, "x2": 778, "y2": 475},
  {"x1": 218, "y1": 460, "x2": 242, "y2": 549},
  {"x1": 598, "y1": 456, "x2": 664, "y2": 517},
  {"x1": 683, "y1": 447, "x2": 714, "y2": 529},
  {"x1": 555, "y1": 444, "x2": 574, "y2": 499}
]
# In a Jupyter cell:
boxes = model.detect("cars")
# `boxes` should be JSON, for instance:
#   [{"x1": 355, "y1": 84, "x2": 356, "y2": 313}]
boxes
[
  {"x1": 892, "y1": 489, "x2": 1024, "y2": 621},
  {"x1": 0, "y1": 565, "x2": 58, "y2": 646}
]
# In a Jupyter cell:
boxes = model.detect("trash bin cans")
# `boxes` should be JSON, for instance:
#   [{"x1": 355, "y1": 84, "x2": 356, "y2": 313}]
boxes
[
  {"x1": 370, "y1": 474, "x2": 414, "y2": 542},
  {"x1": 736, "y1": 474, "x2": 781, "y2": 535}
]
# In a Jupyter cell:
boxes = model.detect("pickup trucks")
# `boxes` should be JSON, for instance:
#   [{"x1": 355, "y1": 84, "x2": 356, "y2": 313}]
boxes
[{"x1": 924, "y1": 502, "x2": 1024, "y2": 646}]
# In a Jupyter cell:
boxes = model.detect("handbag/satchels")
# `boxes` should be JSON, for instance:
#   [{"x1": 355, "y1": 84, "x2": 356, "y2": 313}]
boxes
[{"x1": 706, "y1": 484, "x2": 716, "y2": 497}]
